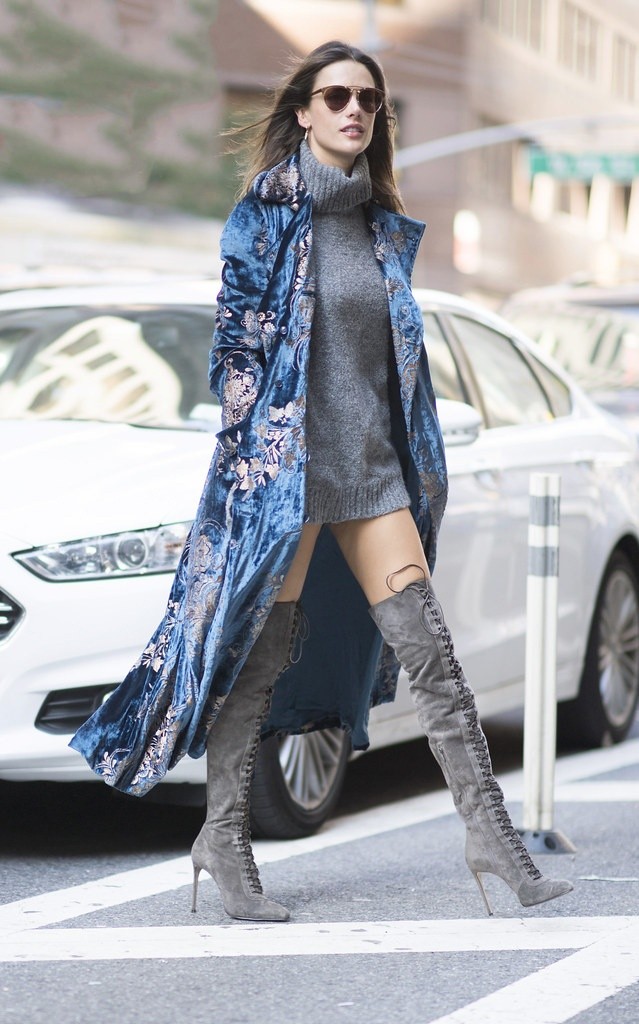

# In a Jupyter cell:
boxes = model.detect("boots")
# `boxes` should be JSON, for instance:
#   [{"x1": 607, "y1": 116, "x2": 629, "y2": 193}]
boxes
[
  {"x1": 190, "y1": 600, "x2": 311, "y2": 921},
  {"x1": 366, "y1": 562, "x2": 574, "y2": 917}
]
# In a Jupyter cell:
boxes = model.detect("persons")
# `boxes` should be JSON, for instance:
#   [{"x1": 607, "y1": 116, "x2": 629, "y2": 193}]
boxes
[{"x1": 67, "y1": 37, "x2": 577, "y2": 922}]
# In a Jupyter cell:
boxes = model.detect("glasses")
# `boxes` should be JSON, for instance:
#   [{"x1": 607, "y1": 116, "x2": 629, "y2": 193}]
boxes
[{"x1": 309, "y1": 84, "x2": 386, "y2": 116}]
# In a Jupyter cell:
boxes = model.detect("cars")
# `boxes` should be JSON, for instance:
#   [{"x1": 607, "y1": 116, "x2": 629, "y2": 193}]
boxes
[{"x1": 0, "y1": 280, "x2": 639, "y2": 834}]
